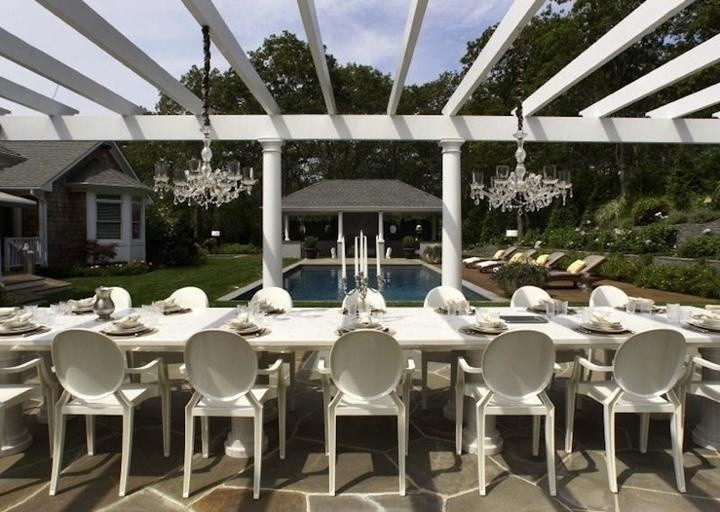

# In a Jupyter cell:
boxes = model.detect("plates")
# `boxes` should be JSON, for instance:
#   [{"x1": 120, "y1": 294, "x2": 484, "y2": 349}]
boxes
[
  {"x1": 501, "y1": 316, "x2": 548, "y2": 323},
  {"x1": 1, "y1": 323, "x2": 41, "y2": 336},
  {"x1": 528, "y1": 304, "x2": 577, "y2": 314},
  {"x1": 258, "y1": 303, "x2": 284, "y2": 316},
  {"x1": 686, "y1": 316, "x2": 720, "y2": 331},
  {"x1": 343, "y1": 324, "x2": 390, "y2": 334},
  {"x1": 343, "y1": 306, "x2": 382, "y2": 314},
  {"x1": 366, "y1": 334, "x2": 367, "y2": 335},
  {"x1": 71, "y1": 305, "x2": 94, "y2": 313},
  {"x1": 220, "y1": 324, "x2": 262, "y2": 334},
  {"x1": 100, "y1": 327, "x2": 150, "y2": 336},
  {"x1": 439, "y1": 307, "x2": 475, "y2": 315},
  {"x1": 155, "y1": 300, "x2": 191, "y2": 315},
  {"x1": 467, "y1": 326, "x2": 509, "y2": 335},
  {"x1": 616, "y1": 303, "x2": 660, "y2": 312},
  {"x1": 580, "y1": 324, "x2": 627, "y2": 333}
]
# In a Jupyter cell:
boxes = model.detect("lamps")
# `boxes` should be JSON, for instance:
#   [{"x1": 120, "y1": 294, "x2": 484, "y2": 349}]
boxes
[
  {"x1": 470, "y1": 47, "x2": 574, "y2": 216},
  {"x1": 152, "y1": 26, "x2": 259, "y2": 211}
]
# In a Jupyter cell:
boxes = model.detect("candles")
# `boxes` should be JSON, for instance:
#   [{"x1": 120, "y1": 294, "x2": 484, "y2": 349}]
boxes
[
  {"x1": 363, "y1": 236, "x2": 368, "y2": 279},
  {"x1": 341, "y1": 235, "x2": 347, "y2": 279},
  {"x1": 354, "y1": 237, "x2": 359, "y2": 276},
  {"x1": 376, "y1": 235, "x2": 381, "y2": 276},
  {"x1": 359, "y1": 229, "x2": 364, "y2": 272}
]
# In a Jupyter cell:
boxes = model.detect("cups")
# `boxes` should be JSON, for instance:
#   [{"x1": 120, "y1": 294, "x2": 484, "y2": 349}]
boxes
[
  {"x1": 489, "y1": 311, "x2": 500, "y2": 324},
  {"x1": 640, "y1": 301, "x2": 653, "y2": 319},
  {"x1": 24, "y1": 301, "x2": 75, "y2": 327},
  {"x1": 447, "y1": 300, "x2": 469, "y2": 325},
  {"x1": 545, "y1": 303, "x2": 556, "y2": 318},
  {"x1": 237, "y1": 300, "x2": 261, "y2": 324},
  {"x1": 141, "y1": 300, "x2": 166, "y2": 327},
  {"x1": 342, "y1": 305, "x2": 384, "y2": 323},
  {"x1": 473, "y1": 308, "x2": 489, "y2": 325},
  {"x1": 666, "y1": 303, "x2": 693, "y2": 325},
  {"x1": 626, "y1": 299, "x2": 637, "y2": 316},
  {"x1": 557, "y1": 301, "x2": 567, "y2": 319}
]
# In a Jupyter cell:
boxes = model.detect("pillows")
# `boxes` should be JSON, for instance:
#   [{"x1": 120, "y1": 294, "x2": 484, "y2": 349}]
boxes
[
  {"x1": 509, "y1": 252, "x2": 525, "y2": 264},
  {"x1": 567, "y1": 259, "x2": 586, "y2": 275},
  {"x1": 494, "y1": 250, "x2": 505, "y2": 260},
  {"x1": 535, "y1": 253, "x2": 550, "y2": 266}
]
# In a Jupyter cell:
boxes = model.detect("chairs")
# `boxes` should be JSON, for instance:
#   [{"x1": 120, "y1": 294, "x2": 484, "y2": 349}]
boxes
[
  {"x1": 165, "y1": 286, "x2": 208, "y2": 307},
  {"x1": 477, "y1": 249, "x2": 538, "y2": 274},
  {"x1": 183, "y1": 328, "x2": 286, "y2": 499},
  {"x1": 464, "y1": 246, "x2": 518, "y2": 269},
  {"x1": 530, "y1": 251, "x2": 566, "y2": 271},
  {"x1": 545, "y1": 255, "x2": 608, "y2": 289},
  {"x1": 92, "y1": 287, "x2": 131, "y2": 307},
  {"x1": 318, "y1": 329, "x2": 416, "y2": 496},
  {"x1": 342, "y1": 286, "x2": 385, "y2": 306},
  {"x1": 423, "y1": 285, "x2": 471, "y2": 410},
  {"x1": 674, "y1": 356, "x2": 720, "y2": 451},
  {"x1": 455, "y1": 329, "x2": 557, "y2": 496},
  {"x1": 565, "y1": 327, "x2": 689, "y2": 494},
  {"x1": 249, "y1": 287, "x2": 296, "y2": 411},
  {"x1": 1, "y1": 357, "x2": 60, "y2": 459},
  {"x1": 48, "y1": 328, "x2": 171, "y2": 497},
  {"x1": 585, "y1": 285, "x2": 630, "y2": 382},
  {"x1": 511, "y1": 285, "x2": 585, "y2": 411}
]
